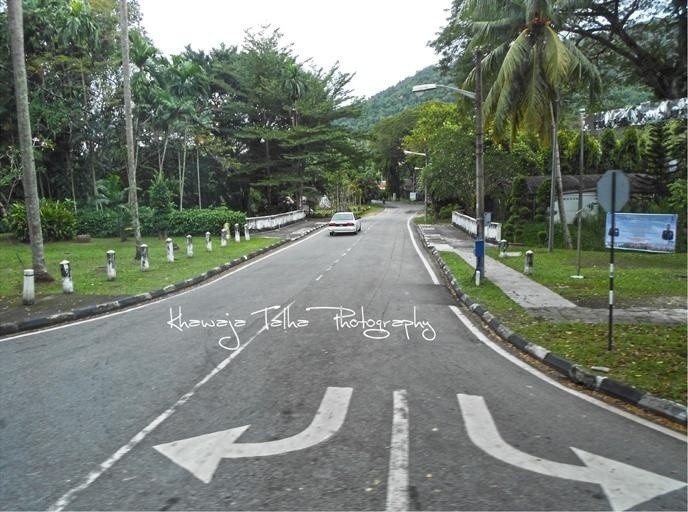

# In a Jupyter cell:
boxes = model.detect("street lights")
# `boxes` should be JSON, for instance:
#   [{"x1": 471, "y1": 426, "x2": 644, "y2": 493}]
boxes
[
  {"x1": 413, "y1": 166, "x2": 425, "y2": 170},
  {"x1": 260, "y1": 137, "x2": 272, "y2": 204},
  {"x1": 411, "y1": 83, "x2": 484, "y2": 281},
  {"x1": 403, "y1": 149, "x2": 428, "y2": 222}
]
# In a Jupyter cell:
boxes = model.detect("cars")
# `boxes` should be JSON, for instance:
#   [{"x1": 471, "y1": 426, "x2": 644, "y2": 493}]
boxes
[{"x1": 328, "y1": 212, "x2": 362, "y2": 236}]
